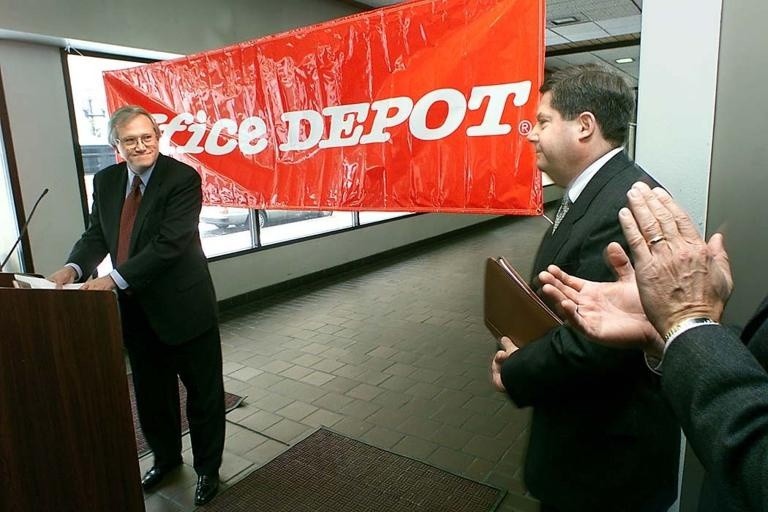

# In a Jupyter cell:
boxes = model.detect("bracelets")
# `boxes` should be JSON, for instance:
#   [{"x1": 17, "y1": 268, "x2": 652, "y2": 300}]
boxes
[{"x1": 663, "y1": 318, "x2": 713, "y2": 343}]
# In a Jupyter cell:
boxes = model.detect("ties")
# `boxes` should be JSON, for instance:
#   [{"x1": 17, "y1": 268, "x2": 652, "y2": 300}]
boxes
[
  {"x1": 115, "y1": 176, "x2": 143, "y2": 269},
  {"x1": 551, "y1": 197, "x2": 571, "y2": 238}
]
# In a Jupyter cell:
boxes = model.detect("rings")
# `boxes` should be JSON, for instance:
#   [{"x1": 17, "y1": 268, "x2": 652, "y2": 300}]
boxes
[{"x1": 647, "y1": 236, "x2": 664, "y2": 246}]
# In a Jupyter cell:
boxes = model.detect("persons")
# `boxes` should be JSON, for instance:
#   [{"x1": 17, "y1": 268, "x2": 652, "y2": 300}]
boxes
[
  {"x1": 538, "y1": 181, "x2": 768, "y2": 512},
  {"x1": 490, "y1": 62, "x2": 680, "y2": 512},
  {"x1": 45, "y1": 104, "x2": 225, "y2": 506}
]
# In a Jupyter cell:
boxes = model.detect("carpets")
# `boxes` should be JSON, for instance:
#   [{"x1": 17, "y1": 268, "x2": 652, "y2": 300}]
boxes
[
  {"x1": 195, "y1": 425, "x2": 509, "y2": 510},
  {"x1": 127, "y1": 370, "x2": 248, "y2": 458}
]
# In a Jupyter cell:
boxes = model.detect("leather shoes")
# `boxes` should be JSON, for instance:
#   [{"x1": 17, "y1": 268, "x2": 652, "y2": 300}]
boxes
[
  {"x1": 195, "y1": 471, "x2": 220, "y2": 505},
  {"x1": 142, "y1": 458, "x2": 181, "y2": 492}
]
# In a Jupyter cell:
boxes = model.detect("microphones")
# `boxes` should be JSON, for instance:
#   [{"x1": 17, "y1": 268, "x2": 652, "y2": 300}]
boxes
[{"x1": 0, "y1": 185, "x2": 52, "y2": 275}]
